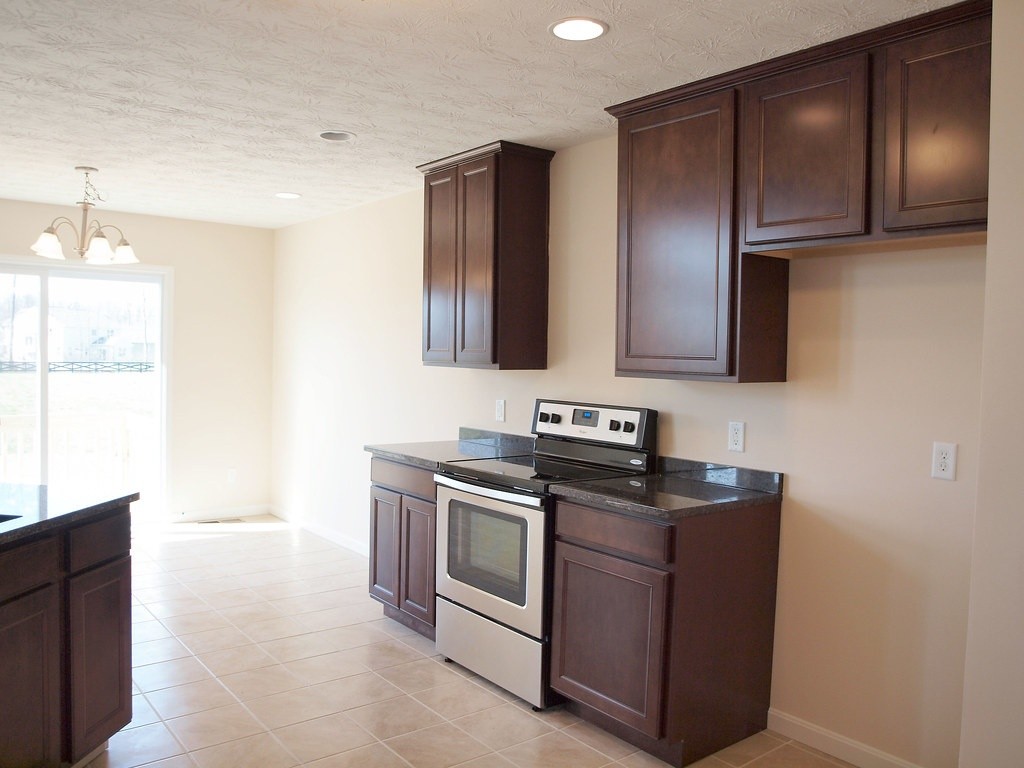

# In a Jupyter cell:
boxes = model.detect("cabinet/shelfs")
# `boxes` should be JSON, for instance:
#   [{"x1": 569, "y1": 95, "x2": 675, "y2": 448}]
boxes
[
  {"x1": 615, "y1": 84, "x2": 790, "y2": 384},
  {"x1": 550, "y1": 499, "x2": 781, "y2": 768},
  {"x1": 0, "y1": 531, "x2": 63, "y2": 768},
  {"x1": 65, "y1": 502, "x2": 133, "y2": 768},
  {"x1": 422, "y1": 152, "x2": 551, "y2": 370},
  {"x1": 741, "y1": 11, "x2": 992, "y2": 261},
  {"x1": 369, "y1": 455, "x2": 436, "y2": 643}
]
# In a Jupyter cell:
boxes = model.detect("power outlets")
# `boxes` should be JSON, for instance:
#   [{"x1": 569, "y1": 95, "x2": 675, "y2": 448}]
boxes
[
  {"x1": 728, "y1": 420, "x2": 745, "y2": 452},
  {"x1": 495, "y1": 399, "x2": 505, "y2": 422},
  {"x1": 931, "y1": 442, "x2": 957, "y2": 481}
]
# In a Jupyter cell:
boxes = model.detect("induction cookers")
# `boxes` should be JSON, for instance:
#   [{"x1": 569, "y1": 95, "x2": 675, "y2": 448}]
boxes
[{"x1": 445, "y1": 399, "x2": 658, "y2": 494}]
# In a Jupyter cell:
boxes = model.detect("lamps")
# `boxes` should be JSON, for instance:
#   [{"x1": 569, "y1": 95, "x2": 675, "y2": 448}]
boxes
[{"x1": 29, "y1": 167, "x2": 139, "y2": 266}]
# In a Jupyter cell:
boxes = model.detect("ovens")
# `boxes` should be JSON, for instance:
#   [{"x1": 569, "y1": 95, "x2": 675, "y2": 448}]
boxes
[{"x1": 432, "y1": 473, "x2": 554, "y2": 714}]
